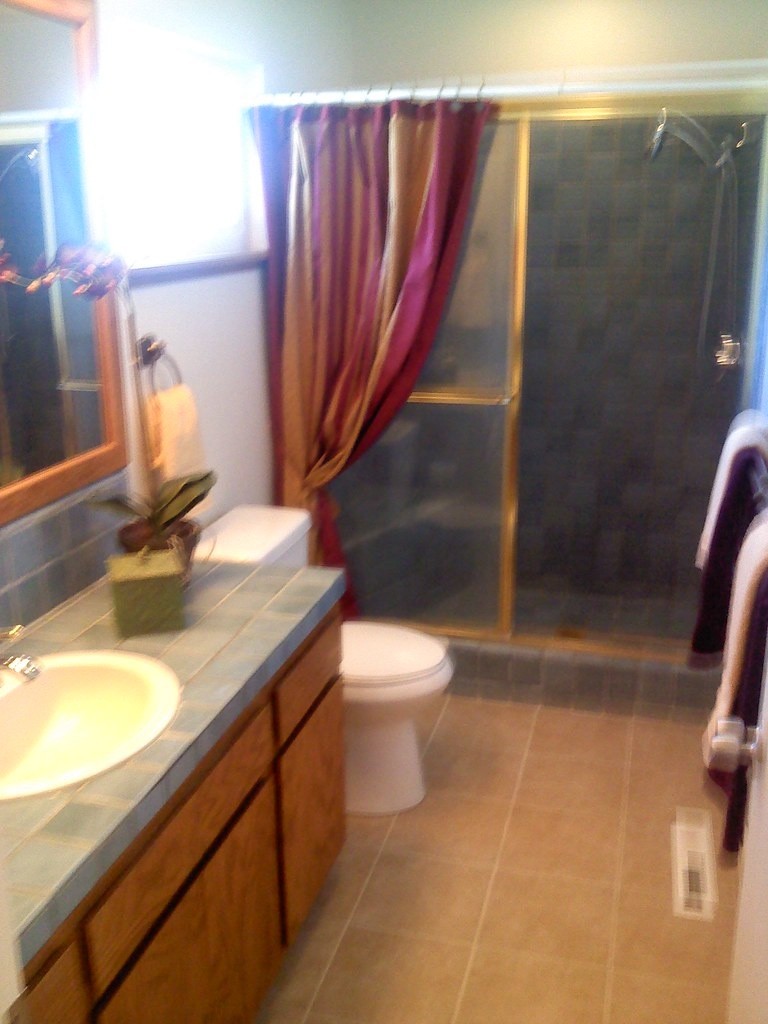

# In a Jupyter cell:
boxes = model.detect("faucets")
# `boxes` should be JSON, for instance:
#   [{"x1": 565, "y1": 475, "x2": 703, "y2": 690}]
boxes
[{"x1": 0, "y1": 623, "x2": 41, "y2": 684}]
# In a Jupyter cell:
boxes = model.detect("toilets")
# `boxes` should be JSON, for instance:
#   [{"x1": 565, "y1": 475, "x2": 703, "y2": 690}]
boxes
[{"x1": 188, "y1": 504, "x2": 455, "y2": 817}]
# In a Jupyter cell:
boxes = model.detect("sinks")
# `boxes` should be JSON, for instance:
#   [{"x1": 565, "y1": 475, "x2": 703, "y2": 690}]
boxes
[{"x1": 1, "y1": 648, "x2": 182, "y2": 802}]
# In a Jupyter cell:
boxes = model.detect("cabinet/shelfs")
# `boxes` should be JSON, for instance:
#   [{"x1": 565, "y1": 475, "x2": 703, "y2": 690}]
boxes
[{"x1": 0, "y1": 607, "x2": 347, "y2": 1024}]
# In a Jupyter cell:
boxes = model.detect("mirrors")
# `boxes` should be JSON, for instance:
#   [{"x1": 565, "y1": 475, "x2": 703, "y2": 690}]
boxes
[{"x1": 0, "y1": 0, "x2": 128, "y2": 528}]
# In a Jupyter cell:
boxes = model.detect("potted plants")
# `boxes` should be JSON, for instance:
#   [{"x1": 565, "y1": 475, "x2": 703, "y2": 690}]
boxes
[{"x1": 93, "y1": 469, "x2": 215, "y2": 564}]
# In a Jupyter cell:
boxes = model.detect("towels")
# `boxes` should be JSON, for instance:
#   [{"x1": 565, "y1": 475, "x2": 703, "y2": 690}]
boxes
[
  {"x1": 684, "y1": 406, "x2": 768, "y2": 870},
  {"x1": 145, "y1": 383, "x2": 198, "y2": 484}
]
